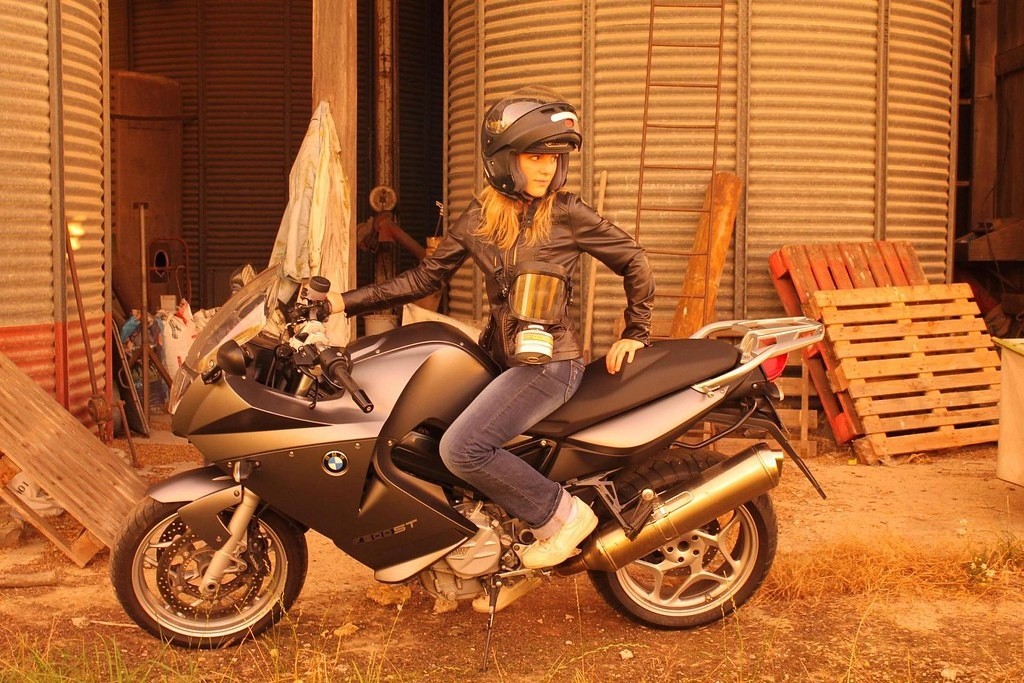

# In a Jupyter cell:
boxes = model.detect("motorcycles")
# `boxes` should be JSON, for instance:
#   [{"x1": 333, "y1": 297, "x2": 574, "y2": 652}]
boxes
[{"x1": 111, "y1": 259, "x2": 830, "y2": 650}]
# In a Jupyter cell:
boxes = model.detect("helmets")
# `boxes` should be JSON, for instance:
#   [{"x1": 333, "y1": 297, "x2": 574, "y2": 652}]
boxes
[{"x1": 480, "y1": 85, "x2": 582, "y2": 200}]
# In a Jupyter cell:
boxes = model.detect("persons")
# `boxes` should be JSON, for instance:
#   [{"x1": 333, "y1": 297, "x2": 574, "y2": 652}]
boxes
[{"x1": 323, "y1": 99, "x2": 655, "y2": 613}]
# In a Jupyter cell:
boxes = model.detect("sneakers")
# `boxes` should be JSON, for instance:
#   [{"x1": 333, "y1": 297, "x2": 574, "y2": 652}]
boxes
[
  {"x1": 473, "y1": 578, "x2": 542, "y2": 613},
  {"x1": 522, "y1": 495, "x2": 598, "y2": 568}
]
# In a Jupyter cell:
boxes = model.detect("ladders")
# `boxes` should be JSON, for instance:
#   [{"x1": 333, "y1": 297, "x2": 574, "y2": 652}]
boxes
[{"x1": 634, "y1": 0, "x2": 725, "y2": 338}]
